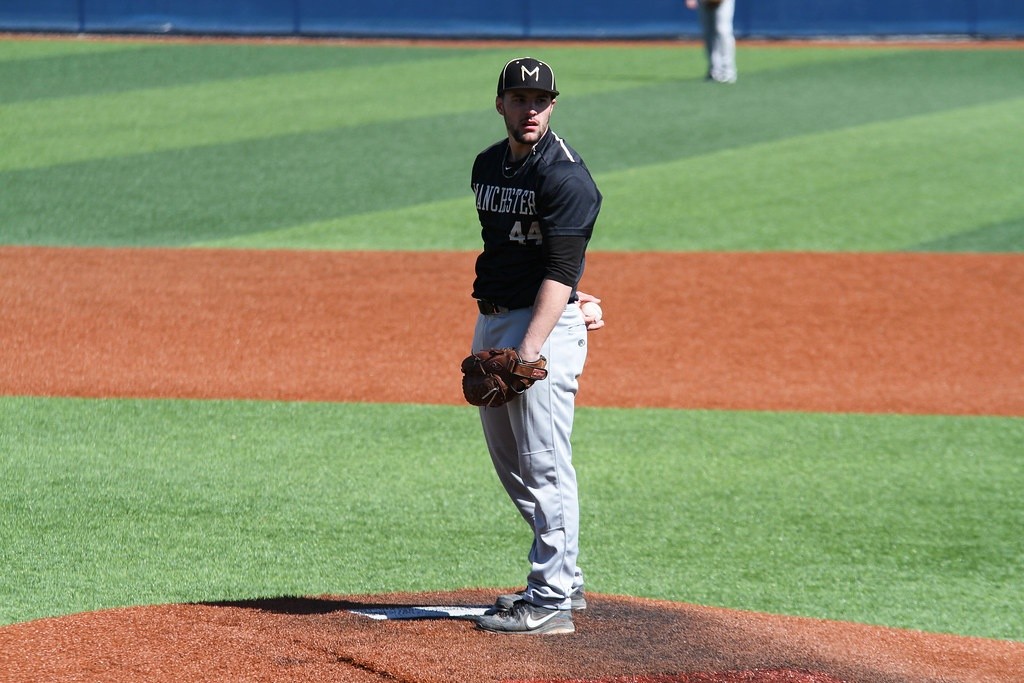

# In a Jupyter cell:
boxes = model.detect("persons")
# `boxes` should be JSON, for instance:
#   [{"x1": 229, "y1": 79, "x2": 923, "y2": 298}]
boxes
[
  {"x1": 686, "y1": 0, "x2": 736, "y2": 84},
  {"x1": 460, "y1": 59, "x2": 604, "y2": 639}
]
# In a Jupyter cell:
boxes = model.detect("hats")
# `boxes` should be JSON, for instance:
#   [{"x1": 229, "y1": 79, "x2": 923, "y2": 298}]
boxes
[{"x1": 497, "y1": 58, "x2": 558, "y2": 99}]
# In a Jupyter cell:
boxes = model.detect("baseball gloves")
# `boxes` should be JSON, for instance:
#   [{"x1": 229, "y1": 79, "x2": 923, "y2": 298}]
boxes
[{"x1": 461, "y1": 349, "x2": 546, "y2": 409}]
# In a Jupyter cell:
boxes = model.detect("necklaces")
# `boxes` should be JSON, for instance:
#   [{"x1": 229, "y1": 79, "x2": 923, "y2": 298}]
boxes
[{"x1": 502, "y1": 144, "x2": 532, "y2": 179}]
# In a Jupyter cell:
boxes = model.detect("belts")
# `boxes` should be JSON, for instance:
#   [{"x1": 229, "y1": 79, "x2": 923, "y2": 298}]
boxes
[{"x1": 477, "y1": 294, "x2": 574, "y2": 315}]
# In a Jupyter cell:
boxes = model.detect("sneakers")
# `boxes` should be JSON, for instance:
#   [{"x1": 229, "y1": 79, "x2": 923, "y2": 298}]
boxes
[
  {"x1": 495, "y1": 585, "x2": 586, "y2": 610},
  {"x1": 475, "y1": 595, "x2": 577, "y2": 634}
]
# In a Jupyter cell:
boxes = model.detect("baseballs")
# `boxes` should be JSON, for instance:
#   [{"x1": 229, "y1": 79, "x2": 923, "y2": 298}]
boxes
[{"x1": 581, "y1": 301, "x2": 602, "y2": 323}]
